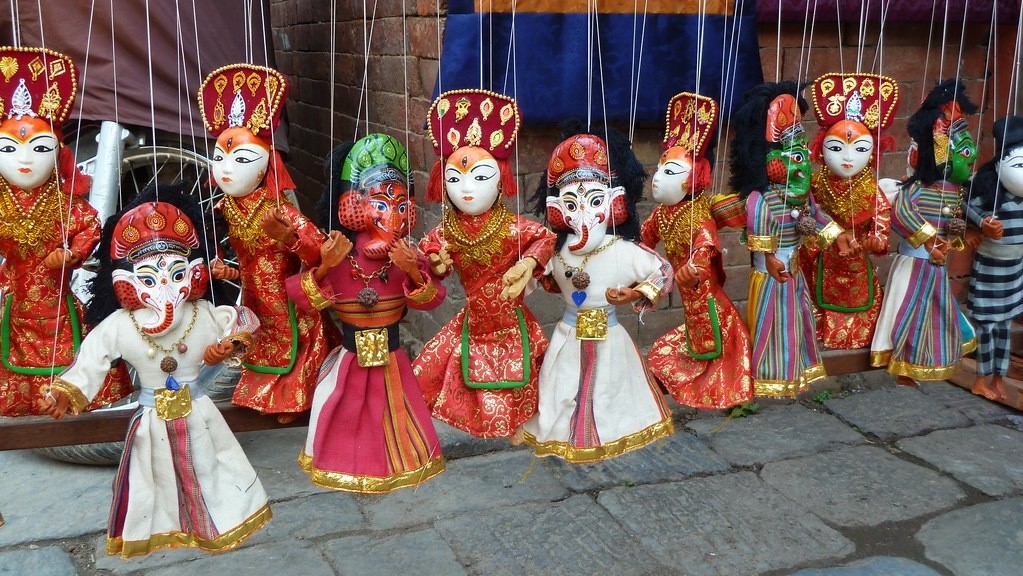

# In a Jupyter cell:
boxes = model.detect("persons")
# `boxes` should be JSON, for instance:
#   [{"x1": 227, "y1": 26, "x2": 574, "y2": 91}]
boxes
[
  {"x1": 0, "y1": 0, "x2": 291, "y2": 329},
  {"x1": 418, "y1": 88, "x2": 558, "y2": 438},
  {"x1": 870, "y1": 78, "x2": 1002, "y2": 389},
  {"x1": 960, "y1": 116, "x2": 1023, "y2": 399},
  {"x1": 0, "y1": 46, "x2": 132, "y2": 418},
  {"x1": 40, "y1": 203, "x2": 274, "y2": 559},
  {"x1": 639, "y1": 91, "x2": 754, "y2": 410},
  {"x1": 512, "y1": 134, "x2": 675, "y2": 464},
  {"x1": 810, "y1": 73, "x2": 901, "y2": 353},
  {"x1": 727, "y1": 82, "x2": 827, "y2": 399},
  {"x1": 198, "y1": 64, "x2": 331, "y2": 425},
  {"x1": 285, "y1": 133, "x2": 447, "y2": 493}
]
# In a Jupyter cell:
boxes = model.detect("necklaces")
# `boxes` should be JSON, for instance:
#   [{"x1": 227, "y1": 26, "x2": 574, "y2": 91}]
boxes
[
  {"x1": 124, "y1": 301, "x2": 197, "y2": 374},
  {"x1": 818, "y1": 164, "x2": 872, "y2": 215},
  {"x1": 345, "y1": 253, "x2": 393, "y2": 307},
  {"x1": 223, "y1": 191, "x2": 266, "y2": 229},
  {"x1": 444, "y1": 203, "x2": 507, "y2": 246},
  {"x1": 555, "y1": 235, "x2": 624, "y2": 290},
  {"x1": 933, "y1": 181, "x2": 966, "y2": 235},
  {"x1": 657, "y1": 189, "x2": 704, "y2": 232},
  {"x1": 0, "y1": 176, "x2": 57, "y2": 218}
]
